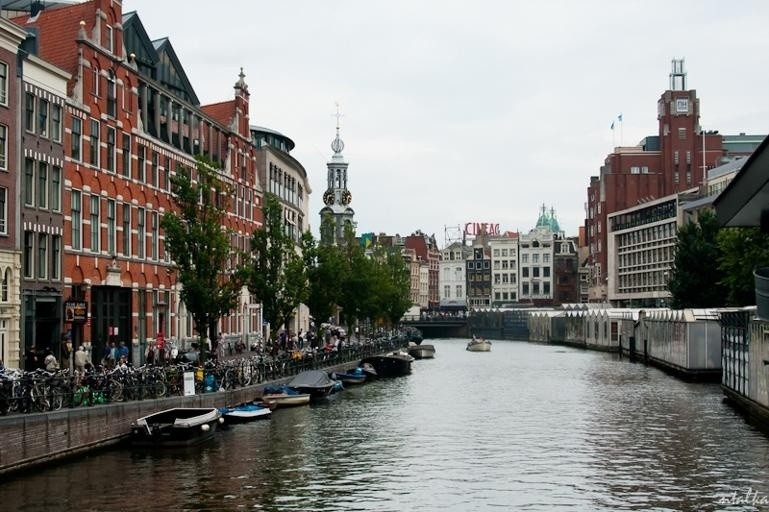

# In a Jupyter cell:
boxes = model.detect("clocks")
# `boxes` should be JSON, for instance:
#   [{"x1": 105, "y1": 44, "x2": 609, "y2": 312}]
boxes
[
  {"x1": 323, "y1": 189, "x2": 335, "y2": 205},
  {"x1": 342, "y1": 190, "x2": 351, "y2": 205}
]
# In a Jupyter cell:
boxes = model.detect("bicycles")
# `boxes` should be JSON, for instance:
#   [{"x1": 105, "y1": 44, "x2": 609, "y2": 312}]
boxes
[
  {"x1": 282, "y1": 334, "x2": 404, "y2": 374},
  {"x1": 0, "y1": 354, "x2": 281, "y2": 414},
  {"x1": 227, "y1": 339, "x2": 246, "y2": 354}
]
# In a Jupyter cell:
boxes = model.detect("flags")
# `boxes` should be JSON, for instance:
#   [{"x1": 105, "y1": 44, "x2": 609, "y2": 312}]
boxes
[
  {"x1": 611, "y1": 122, "x2": 614, "y2": 129},
  {"x1": 619, "y1": 114, "x2": 622, "y2": 122}
]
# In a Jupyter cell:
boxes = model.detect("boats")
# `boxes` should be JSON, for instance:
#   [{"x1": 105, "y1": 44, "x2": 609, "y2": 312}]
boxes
[
  {"x1": 288, "y1": 369, "x2": 339, "y2": 395},
  {"x1": 219, "y1": 400, "x2": 273, "y2": 419},
  {"x1": 408, "y1": 345, "x2": 435, "y2": 359},
  {"x1": 467, "y1": 340, "x2": 491, "y2": 352},
  {"x1": 363, "y1": 349, "x2": 414, "y2": 374},
  {"x1": 259, "y1": 381, "x2": 312, "y2": 406},
  {"x1": 341, "y1": 360, "x2": 368, "y2": 384},
  {"x1": 132, "y1": 407, "x2": 225, "y2": 448}
]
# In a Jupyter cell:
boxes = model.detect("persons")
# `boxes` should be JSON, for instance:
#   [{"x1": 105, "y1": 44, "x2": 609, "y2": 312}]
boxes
[
  {"x1": 421, "y1": 310, "x2": 453, "y2": 320},
  {"x1": 24, "y1": 320, "x2": 346, "y2": 372}
]
